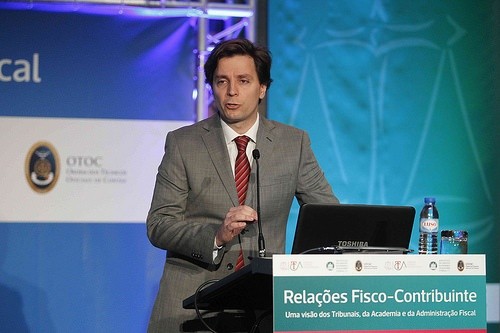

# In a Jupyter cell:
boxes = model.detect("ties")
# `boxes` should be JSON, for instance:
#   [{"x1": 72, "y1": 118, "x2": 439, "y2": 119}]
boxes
[{"x1": 231, "y1": 136, "x2": 251, "y2": 271}]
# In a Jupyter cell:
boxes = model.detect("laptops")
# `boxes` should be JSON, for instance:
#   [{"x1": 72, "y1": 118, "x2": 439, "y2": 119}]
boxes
[{"x1": 290, "y1": 203, "x2": 416, "y2": 254}]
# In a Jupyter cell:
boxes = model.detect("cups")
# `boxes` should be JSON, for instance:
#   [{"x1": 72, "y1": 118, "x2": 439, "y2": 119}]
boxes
[
  {"x1": 440, "y1": 229, "x2": 455, "y2": 255},
  {"x1": 456, "y1": 231, "x2": 468, "y2": 255}
]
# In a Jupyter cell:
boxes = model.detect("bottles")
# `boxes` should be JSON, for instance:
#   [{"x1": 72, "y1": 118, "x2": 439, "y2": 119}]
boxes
[{"x1": 418, "y1": 197, "x2": 440, "y2": 254}]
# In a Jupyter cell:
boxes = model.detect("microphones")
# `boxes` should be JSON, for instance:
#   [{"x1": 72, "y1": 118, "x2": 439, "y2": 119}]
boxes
[{"x1": 252, "y1": 149, "x2": 266, "y2": 257}]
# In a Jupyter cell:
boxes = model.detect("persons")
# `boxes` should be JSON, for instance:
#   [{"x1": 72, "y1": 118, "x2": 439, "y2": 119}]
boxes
[{"x1": 146, "y1": 38, "x2": 341, "y2": 333}]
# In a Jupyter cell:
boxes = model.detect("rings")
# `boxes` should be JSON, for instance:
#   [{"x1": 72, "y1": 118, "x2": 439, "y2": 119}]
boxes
[{"x1": 226, "y1": 224, "x2": 233, "y2": 232}]
